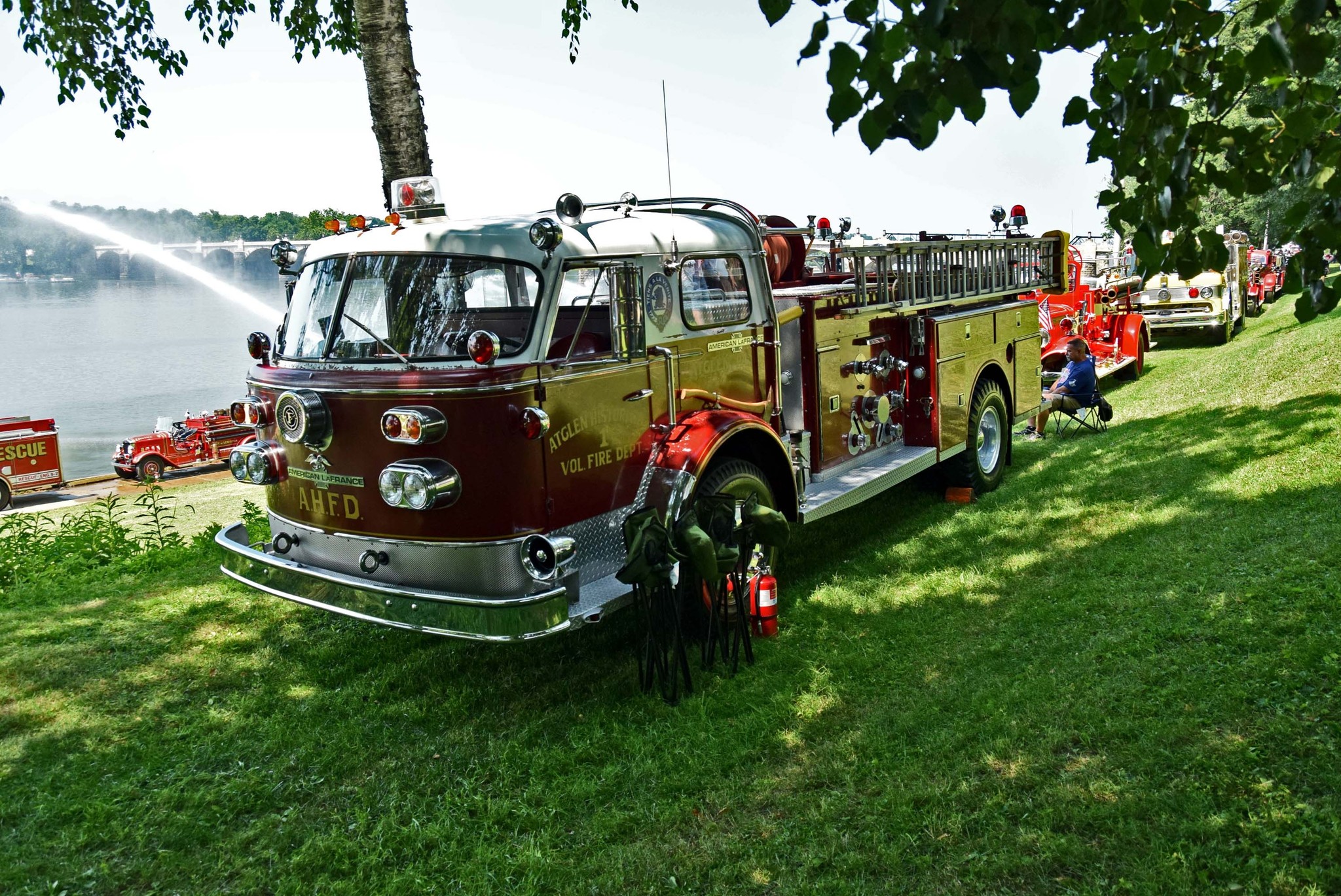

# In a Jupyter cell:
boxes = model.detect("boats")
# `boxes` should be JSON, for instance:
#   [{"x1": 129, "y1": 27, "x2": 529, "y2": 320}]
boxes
[
  {"x1": 0, "y1": 272, "x2": 24, "y2": 283},
  {"x1": 22, "y1": 274, "x2": 49, "y2": 283},
  {"x1": 51, "y1": 274, "x2": 74, "y2": 283}
]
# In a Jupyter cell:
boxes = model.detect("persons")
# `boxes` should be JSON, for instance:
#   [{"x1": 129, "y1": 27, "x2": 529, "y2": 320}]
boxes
[{"x1": 1013, "y1": 338, "x2": 1096, "y2": 441}]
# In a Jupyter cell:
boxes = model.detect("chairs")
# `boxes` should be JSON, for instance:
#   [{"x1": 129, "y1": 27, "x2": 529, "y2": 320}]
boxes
[
  {"x1": 1043, "y1": 354, "x2": 1107, "y2": 439},
  {"x1": 615, "y1": 490, "x2": 792, "y2": 703}
]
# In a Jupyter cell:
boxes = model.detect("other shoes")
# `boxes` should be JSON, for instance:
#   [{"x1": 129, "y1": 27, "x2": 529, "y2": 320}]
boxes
[
  {"x1": 1023, "y1": 433, "x2": 1045, "y2": 441},
  {"x1": 1013, "y1": 427, "x2": 1035, "y2": 436}
]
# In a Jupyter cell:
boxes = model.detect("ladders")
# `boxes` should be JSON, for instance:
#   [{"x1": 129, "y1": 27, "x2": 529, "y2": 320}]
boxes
[{"x1": 830, "y1": 230, "x2": 1070, "y2": 319}]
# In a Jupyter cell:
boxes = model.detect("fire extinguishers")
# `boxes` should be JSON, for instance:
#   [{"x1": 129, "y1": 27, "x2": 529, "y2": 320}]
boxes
[{"x1": 744, "y1": 556, "x2": 778, "y2": 638}]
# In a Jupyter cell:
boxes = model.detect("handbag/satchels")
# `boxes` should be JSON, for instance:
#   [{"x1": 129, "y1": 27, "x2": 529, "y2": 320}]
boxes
[{"x1": 1099, "y1": 397, "x2": 1113, "y2": 421}]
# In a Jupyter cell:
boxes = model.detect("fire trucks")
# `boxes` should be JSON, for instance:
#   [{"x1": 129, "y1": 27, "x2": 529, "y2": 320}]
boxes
[
  {"x1": 212, "y1": 179, "x2": 1069, "y2": 644},
  {"x1": 1014, "y1": 223, "x2": 1304, "y2": 392},
  {"x1": 111, "y1": 409, "x2": 258, "y2": 482},
  {"x1": 0, "y1": 417, "x2": 67, "y2": 513}
]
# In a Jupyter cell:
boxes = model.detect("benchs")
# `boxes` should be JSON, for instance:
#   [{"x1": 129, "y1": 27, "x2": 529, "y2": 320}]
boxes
[{"x1": 426, "y1": 307, "x2": 612, "y2": 360}]
